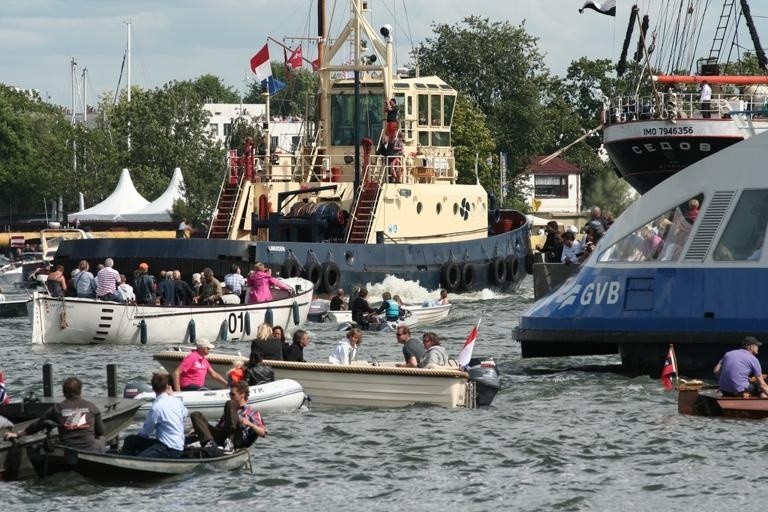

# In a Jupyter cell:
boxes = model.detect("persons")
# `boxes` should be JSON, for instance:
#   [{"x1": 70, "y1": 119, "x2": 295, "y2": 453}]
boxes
[
  {"x1": 713, "y1": 335, "x2": 768, "y2": 398},
  {"x1": 4, "y1": 377, "x2": 106, "y2": 454},
  {"x1": 373, "y1": 97, "x2": 405, "y2": 183},
  {"x1": 193, "y1": 380, "x2": 266, "y2": 458},
  {"x1": 255, "y1": 113, "x2": 305, "y2": 122},
  {"x1": 698, "y1": 79, "x2": 712, "y2": 118},
  {"x1": 535, "y1": 197, "x2": 766, "y2": 266},
  {"x1": 123, "y1": 372, "x2": 189, "y2": 458}
]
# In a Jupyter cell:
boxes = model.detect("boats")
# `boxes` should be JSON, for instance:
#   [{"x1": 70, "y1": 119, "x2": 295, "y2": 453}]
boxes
[
  {"x1": 512, "y1": 125, "x2": 767, "y2": 368},
  {"x1": 152, "y1": 343, "x2": 500, "y2": 411},
  {"x1": 309, "y1": 297, "x2": 459, "y2": 329},
  {"x1": 55, "y1": 0, "x2": 534, "y2": 302},
  {"x1": 340, "y1": 311, "x2": 423, "y2": 336},
  {"x1": 665, "y1": 343, "x2": 768, "y2": 417},
  {"x1": 0, "y1": 216, "x2": 99, "y2": 311},
  {"x1": 533, "y1": 0, "x2": 768, "y2": 302},
  {"x1": 26, "y1": 271, "x2": 312, "y2": 344}
]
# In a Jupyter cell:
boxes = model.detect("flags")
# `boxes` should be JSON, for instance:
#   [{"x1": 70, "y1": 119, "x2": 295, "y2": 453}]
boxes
[
  {"x1": 288, "y1": 46, "x2": 302, "y2": 68},
  {"x1": 455, "y1": 328, "x2": 479, "y2": 371},
  {"x1": 661, "y1": 347, "x2": 675, "y2": 391},
  {"x1": 250, "y1": 43, "x2": 287, "y2": 94},
  {"x1": 579, "y1": 0, "x2": 615, "y2": 16}
]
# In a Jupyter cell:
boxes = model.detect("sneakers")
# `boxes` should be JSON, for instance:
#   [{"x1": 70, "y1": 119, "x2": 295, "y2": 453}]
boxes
[{"x1": 223, "y1": 438, "x2": 234, "y2": 454}]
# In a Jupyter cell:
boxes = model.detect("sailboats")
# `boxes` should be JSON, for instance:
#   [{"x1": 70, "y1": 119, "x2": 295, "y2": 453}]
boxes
[{"x1": 2, "y1": 20, "x2": 133, "y2": 230}]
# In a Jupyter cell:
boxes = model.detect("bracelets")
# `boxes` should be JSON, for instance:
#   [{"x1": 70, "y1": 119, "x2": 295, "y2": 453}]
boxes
[{"x1": 16, "y1": 432, "x2": 19, "y2": 439}]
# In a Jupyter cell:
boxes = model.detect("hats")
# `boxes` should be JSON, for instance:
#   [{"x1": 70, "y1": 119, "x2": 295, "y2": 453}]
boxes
[
  {"x1": 197, "y1": 340, "x2": 215, "y2": 348},
  {"x1": 139, "y1": 263, "x2": 149, "y2": 270}
]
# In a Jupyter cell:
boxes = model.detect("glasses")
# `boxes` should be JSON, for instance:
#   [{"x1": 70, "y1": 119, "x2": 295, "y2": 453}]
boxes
[{"x1": 397, "y1": 333, "x2": 401, "y2": 336}]
